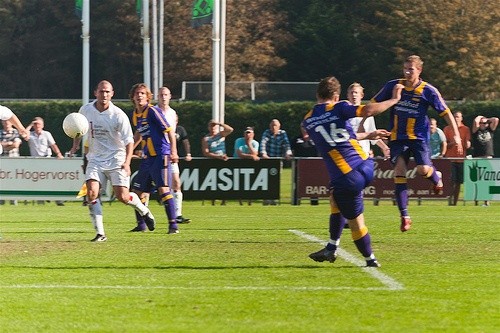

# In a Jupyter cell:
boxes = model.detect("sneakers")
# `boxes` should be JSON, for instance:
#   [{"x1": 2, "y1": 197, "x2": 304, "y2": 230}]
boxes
[
  {"x1": 141, "y1": 211, "x2": 155, "y2": 231},
  {"x1": 401, "y1": 218, "x2": 411, "y2": 231},
  {"x1": 91, "y1": 234, "x2": 107, "y2": 241},
  {"x1": 434, "y1": 171, "x2": 444, "y2": 195},
  {"x1": 370, "y1": 261, "x2": 380, "y2": 267},
  {"x1": 308, "y1": 247, "x2": 337, "y2": 263}
]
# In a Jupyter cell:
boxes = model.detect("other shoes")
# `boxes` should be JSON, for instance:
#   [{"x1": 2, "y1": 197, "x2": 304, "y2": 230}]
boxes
[
  {"x1": 176, "y1": 216, "x2": 192, "y2": 224},
  {"x1": 167, "y1": 229, "x2": 178, "y2": 235},
  {"x1": 130, "y1": 227, "x2": 142, "y2": 232}
]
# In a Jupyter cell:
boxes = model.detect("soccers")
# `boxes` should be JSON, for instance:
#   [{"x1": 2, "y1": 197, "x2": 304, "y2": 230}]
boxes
[{"x1": 63, "y1": 112, "x2": 89, "y2": 138}]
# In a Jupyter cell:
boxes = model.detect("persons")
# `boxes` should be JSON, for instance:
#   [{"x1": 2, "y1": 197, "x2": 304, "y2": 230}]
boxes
[
  {"x1": 175, "y1": 125, "x2": 192, "y2": 161},
  {"x1": 127, "y1": 83, "x2": 179, "y2": 235},
  {"x1": 233, "y1": 127, "x2": 259, "y2": 161},
  {"x1": 133, "y1": 130, "x2": 147, "y2": 159},
  {"x1": 472, "y1": 115, "x2": 499, "y2": 206},
  {"x1": 300, "y1": 76, "x2": 405, "y2": 267},
  {"x1": 259, "y1": 119, "x2": 293, "y2": 160},
  {"x1": 356, "y1": 55, "x2": 463, "y2": 232},
  {"x1": 201, "y1": 120, "x2": 233, "y2": 162},
  {"x1": 443, "y1": 112, "x2": 471, "y2": 205},
  {"x1": 343, "y1": 82, "x2": 390, "y2": 229},
  {"x1": 0, "y1": 104, "x2": 64, "y2": 159},
  {"x1": 411, "y1": 118, "x2": 447, "y2": 195},
  {"x1": 145, "y1": 87, "x2": 191, "y2": 224},
  {"x1": 69, "y1": 79, "x2": 156, "y2": 242}
]
